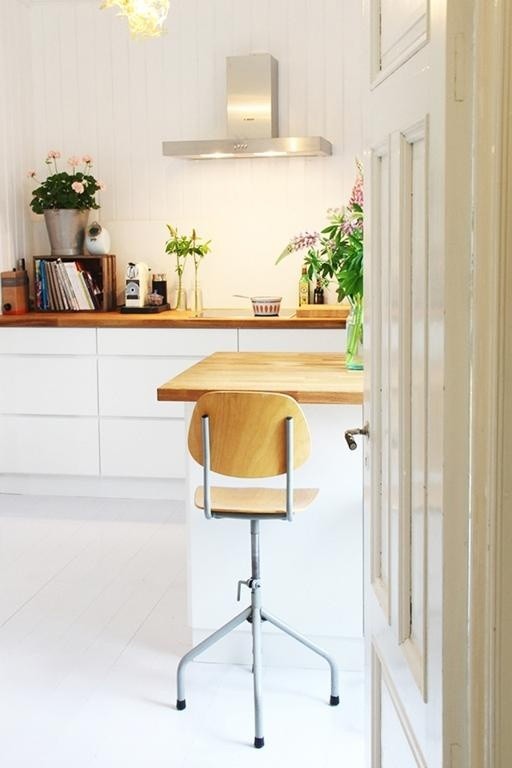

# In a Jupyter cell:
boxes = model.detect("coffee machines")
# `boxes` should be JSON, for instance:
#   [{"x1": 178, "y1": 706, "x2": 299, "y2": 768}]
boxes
[{"x1": 125, "y1": 263, "x2": 152, "y2": 307}]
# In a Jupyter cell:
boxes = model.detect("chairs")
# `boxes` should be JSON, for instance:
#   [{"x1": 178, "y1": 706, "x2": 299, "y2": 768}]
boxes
[{"x1": 174, "y1": 391, "x2": 340, "y2": 750}]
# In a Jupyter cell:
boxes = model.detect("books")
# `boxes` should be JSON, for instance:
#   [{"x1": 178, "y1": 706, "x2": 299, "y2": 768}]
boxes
[{"x1": 34, "y1": 259, "x2": 99, "y2": 311}]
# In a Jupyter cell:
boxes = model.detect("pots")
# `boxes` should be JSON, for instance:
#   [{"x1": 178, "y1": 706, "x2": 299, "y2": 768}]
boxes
[{"x1": 233, "y1": 293, "x2": 282, "y2": 317}]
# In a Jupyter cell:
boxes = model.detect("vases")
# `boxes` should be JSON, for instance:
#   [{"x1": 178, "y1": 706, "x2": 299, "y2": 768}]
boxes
[
  {"x1": 44, "y1": 207, "x2": 84, "y2": 256},
  {"x1": 347, "y1": 303, "x2": 362, "y2": 366}
]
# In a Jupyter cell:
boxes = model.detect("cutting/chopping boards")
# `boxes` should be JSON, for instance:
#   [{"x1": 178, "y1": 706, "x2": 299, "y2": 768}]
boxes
[{"x1": 296, "y1": 303, "x2": 352, "y2": 318}]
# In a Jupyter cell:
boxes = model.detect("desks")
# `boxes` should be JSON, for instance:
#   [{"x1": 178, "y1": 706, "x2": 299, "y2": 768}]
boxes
[{"x1": 158, "y1": 352, "x2": 367, "y2": 410}]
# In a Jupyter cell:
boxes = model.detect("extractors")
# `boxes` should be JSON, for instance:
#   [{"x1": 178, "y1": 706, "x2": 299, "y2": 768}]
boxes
[{"x1": 162, "y1": 53, "x2": 333, "y2": 158}]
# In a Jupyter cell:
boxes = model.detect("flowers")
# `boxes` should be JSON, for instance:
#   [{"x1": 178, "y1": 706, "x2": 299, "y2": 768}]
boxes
[
  {"x1": 26, "y1": 150, "x2": 101, "y2": 215},
  {"x1": 163, "y1": 221, "x2": 191, "y2": 304},
  {"x1": 274, "y1": 154, "x2": 363, "y2": 354},
  {"x1": 187, "y1": 231, "x2": 214, "y2": 318}
]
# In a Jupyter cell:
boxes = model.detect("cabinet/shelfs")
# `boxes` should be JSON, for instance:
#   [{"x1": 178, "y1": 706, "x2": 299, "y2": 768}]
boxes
[
  {"x1": 0, "y1": 327, "x2": 99, "y2": 504},
  {"x1": 98, "y1": 327, "x2": 237, "y2": 502},
  {"x1": 238, "y1": 329, "x2": 360, "y2": 514}
]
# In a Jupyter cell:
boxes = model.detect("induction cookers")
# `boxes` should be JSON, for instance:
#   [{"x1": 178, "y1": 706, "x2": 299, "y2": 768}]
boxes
[{"x1": 193, "y1": 308, "x2": 293, "y2": 318}]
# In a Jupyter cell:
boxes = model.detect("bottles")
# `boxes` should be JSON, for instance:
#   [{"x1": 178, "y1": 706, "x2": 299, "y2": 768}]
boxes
[
  {"x1": 314, "y1": 278, "x2": 323, "y2": 304},
  {"x1": 152, "y1": 272, "x2": 167, "y2": 305},
  {"x1": 299, "y1": 264, "x2": 311, "y2": 307}
]
